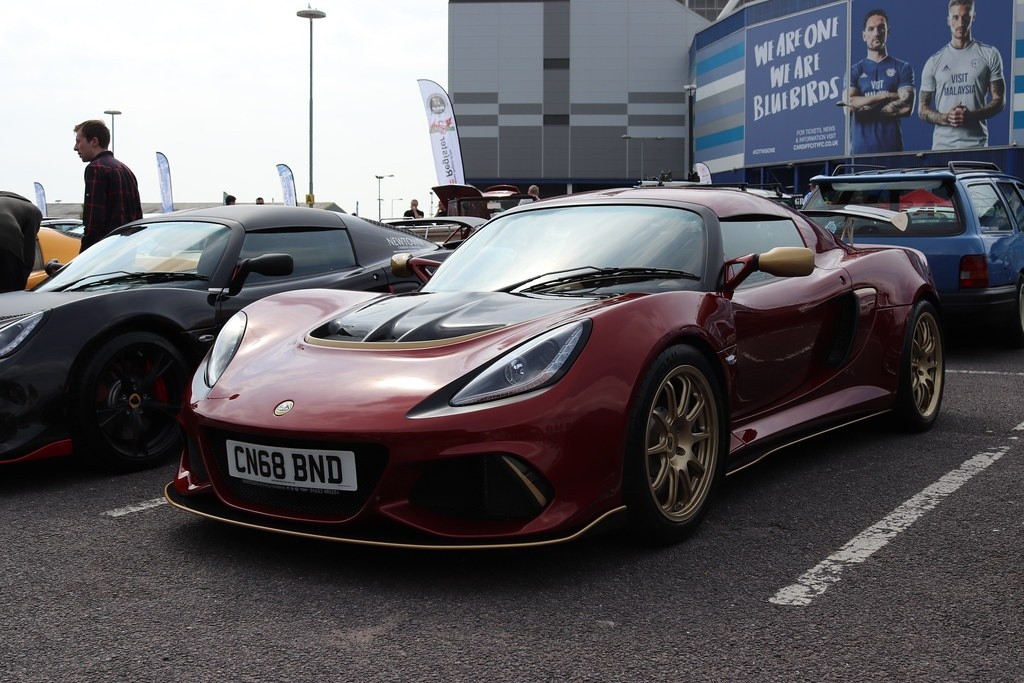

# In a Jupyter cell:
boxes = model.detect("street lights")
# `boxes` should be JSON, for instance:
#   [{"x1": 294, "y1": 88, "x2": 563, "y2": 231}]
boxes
[
  {"x1": 378, "y1": 175, "x2": 394, "y2": 222},
  {"x1": 391, "y1": 198, "x2": 403, "y2": 217},
  {"x1": 296, "y1": 3, "x2": 326, "y2": 209},
  {"x1": 430, "y1": 192, "x2": 433, "y2": 217},
  {"x1": 104, "y1": 110, "x2": 122, "y2": 159}
]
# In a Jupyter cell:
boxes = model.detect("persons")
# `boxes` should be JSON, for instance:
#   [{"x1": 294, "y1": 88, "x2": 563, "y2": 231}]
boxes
[
  {"x1": 518, "y1": 185, "x2": 541, "y2": 206},
  {"x1": 841, "y1": 9, "x2": 915, "y2": 155},
  {"x1": 917, "y1": 0, "x2": 1006, "y2": 151},
  {"x1": 73, "y1": 120, "x2": 143, "y2": 254},
  {"x1": 225, "y1": 195, "x2": 236, "y2": 205},
  {"x1": 404, "y1": 200, "x2": 424, "y2": 219},
  {"x1": 435, "y1": 201, "x2": 447, "y2": 225},
  {"x1": 803, "y1": 175, "x2": 825, "y2": 205},
  {"x1": 256, "y1": 197, "x2": 264, "y2": 204},
  {"x1": 0, "y1": 191, "x2": 43, "y2": 293}
]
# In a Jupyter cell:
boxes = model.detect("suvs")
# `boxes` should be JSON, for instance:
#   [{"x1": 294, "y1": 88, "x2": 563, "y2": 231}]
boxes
[
  {"x1": 634, "y1": 170, "x2": 797, "y2": 209},
  {"x1": 799, "y1": 161, "x2": 1024, "y2": 335}
]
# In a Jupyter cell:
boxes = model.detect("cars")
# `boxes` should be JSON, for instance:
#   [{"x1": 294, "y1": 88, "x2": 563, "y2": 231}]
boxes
[{"x1": 387, "y1": 216, "x2": 488, "y2": 242}]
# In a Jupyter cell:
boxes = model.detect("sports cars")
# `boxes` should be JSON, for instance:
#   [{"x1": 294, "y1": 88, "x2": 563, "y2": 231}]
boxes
[
  {"x1": 164, "y1": 187, "x2": 947, "y2": 550},
  {"x1": 1, "y1": 204, "x2": 453, "y2": 474}
]
